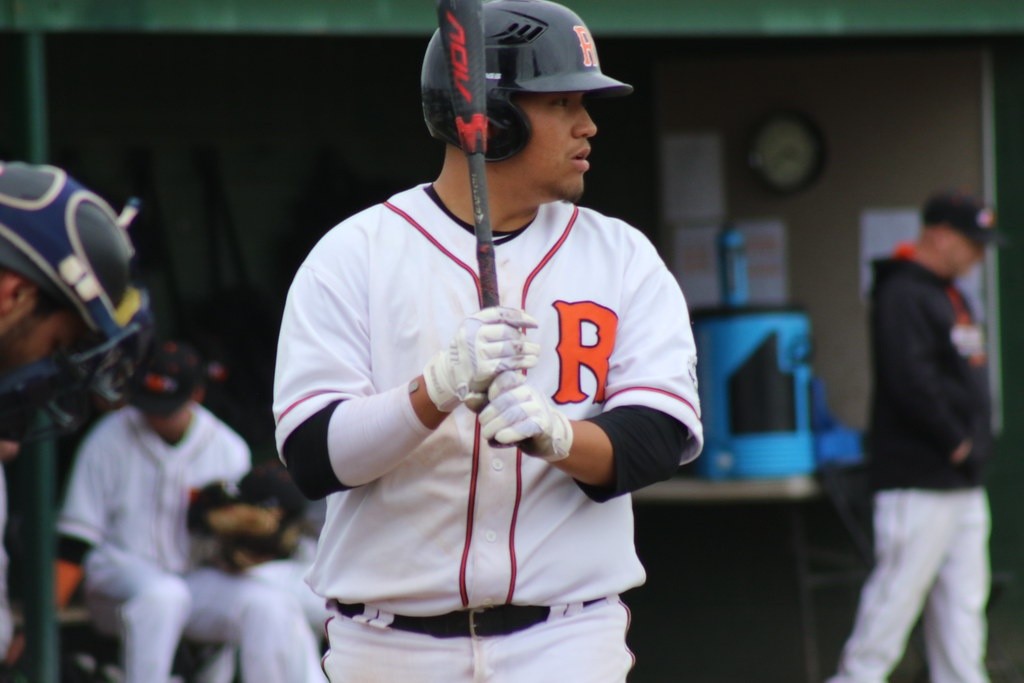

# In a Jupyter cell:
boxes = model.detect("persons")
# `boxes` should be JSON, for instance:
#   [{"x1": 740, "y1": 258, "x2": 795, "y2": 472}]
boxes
[
  {"x1": 0, "y1": 161, "x2": 323, "y2": 682},
  {"x1": 826, "y1": 191, "x2": 1011, "y2": 683},
  {"x1": 273, "y1": 0, "x2": 705, "y2": 682}
]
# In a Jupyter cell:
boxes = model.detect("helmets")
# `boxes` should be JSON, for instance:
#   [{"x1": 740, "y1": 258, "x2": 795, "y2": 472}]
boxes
[
  {"x1": 419, "y1": 0, "x2": 633, "y2": 161},
  {"x1": 0, "y1": 162, "x2": 148, "y2": 350}
]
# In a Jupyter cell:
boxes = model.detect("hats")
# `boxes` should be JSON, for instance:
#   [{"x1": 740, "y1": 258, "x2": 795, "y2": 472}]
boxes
[
  {"x1": 922, "y1": 194, "x2": 1011, "y2": 251},
  {"x1": 117, "y1": 341, "x2": 208, "y2": 419}
]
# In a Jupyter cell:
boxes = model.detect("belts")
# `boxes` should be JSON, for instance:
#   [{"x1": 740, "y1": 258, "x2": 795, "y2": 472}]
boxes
[{"x1": 331, "y1": 595, "x2": 608, "y2": 638}]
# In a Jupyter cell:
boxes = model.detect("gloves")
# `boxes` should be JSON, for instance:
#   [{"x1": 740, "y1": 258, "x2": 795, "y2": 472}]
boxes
[
  {"x1": 465, "y1": 370, "x2": 573, "y2": 463},
  {"x1": 423, "y1": 306, "x2": 539, "y2": 412}
]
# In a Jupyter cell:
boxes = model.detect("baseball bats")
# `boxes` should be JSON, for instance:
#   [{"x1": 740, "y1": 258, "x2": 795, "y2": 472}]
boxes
[{"x1": 435, "y1": 1, "x2": 521, "y2": 451}]
